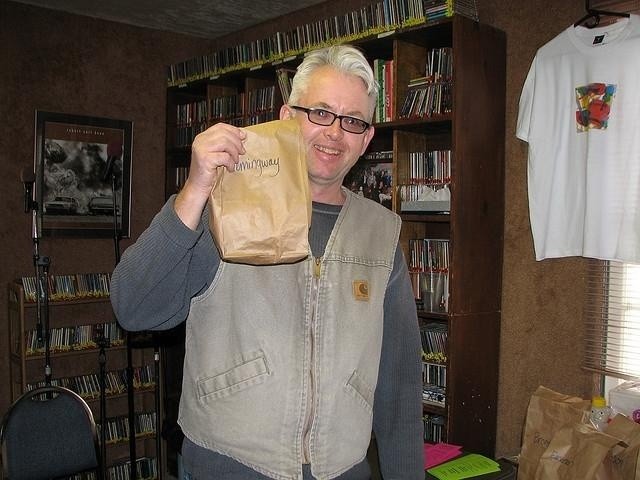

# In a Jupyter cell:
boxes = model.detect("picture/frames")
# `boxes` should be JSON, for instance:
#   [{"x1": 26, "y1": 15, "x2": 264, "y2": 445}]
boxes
[{"x1": 32, "y1": 107, "x2": 135, "y2": 240}]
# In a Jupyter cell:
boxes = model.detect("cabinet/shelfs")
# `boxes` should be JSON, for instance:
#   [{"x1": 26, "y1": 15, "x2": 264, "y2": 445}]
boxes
[
  {"x1": 162, "y1": 15, "x2": 507, "y2": 461},
  {"x1": 8, "y1": 279, "x2": 162, "y2": 480}
]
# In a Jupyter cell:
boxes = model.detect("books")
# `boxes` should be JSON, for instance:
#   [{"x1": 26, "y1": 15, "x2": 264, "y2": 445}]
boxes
[
  {"x1": 421, "y1": 362, "x2": 447, "y2": 408},
  {"x1": 176, "y1": 166, "x2": 189, "y2": 195},
  {"x1": 167, "y1": 0, "x2": 481, "y2": 83},
  {"x1": 421, "y1": 322, "x2": 447, "y2": 365},
  {"x1": 422, "y1": 412, "x2": 448, "y2": 441},
  {"x1": 175, "y1": 68, "x2": 298, "y2": 148},
  {"x1": 397, "y1": 48, "x2": 453, "y2": 118},
  {"x1": 370, "y1": 58, "x2": 396, "y2": 124},
  {"x1": 408, "y1": 240, "x2": 448, "y2": 312},
  {"x1": 401, "y1": 150, "x2": 451, "y2": 202},
  {"x1": 19, "y1": 272, "x2": 159, "y2": 480}
]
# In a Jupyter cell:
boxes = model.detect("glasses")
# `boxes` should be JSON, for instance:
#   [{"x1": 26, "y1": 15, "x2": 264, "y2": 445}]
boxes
[{"x1": 291, "y1": 106, "x2": 372, "y2": 135}]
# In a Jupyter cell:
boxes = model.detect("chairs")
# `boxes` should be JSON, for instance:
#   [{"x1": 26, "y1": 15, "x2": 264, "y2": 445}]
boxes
[{"x1": 1, "y1": 384, "x2": 104, "y2": 480}]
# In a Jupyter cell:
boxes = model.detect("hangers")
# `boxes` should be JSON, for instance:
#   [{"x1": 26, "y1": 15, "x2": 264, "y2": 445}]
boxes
[{"x1": 574, "y1": 0, "x2": 630, "y2": 29}]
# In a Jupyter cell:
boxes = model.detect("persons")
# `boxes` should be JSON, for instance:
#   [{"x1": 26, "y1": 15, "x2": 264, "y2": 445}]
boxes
[
  {"x1": 108, "y1": 44, "x2": 428, "y2": 480},
  {"x1": 345, "y1": 168, "x2": 393, "y2": 205}
]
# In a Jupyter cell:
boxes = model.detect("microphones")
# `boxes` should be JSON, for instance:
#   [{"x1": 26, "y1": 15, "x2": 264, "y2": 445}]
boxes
[
  {"x1": 101, "y1": 142, "x2": 123, "y2": 179},
  {"x1": 21, "y1": 166, "x2": 34, "y2": 212}
]
